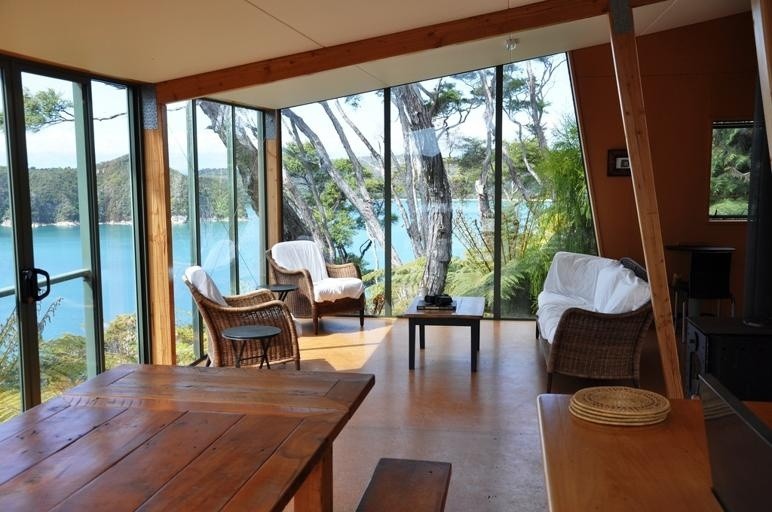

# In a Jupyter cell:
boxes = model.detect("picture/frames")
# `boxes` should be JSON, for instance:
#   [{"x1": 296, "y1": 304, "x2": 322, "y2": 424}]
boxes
[
  {"x1": 254, "y1": 284, "x2": 299, "y2": 305},
  {"x1": 220, "y1": 324, "x2": 283, "y2": 371},
  {"x1": 0, "y1": 360, "x2": 378, "y2": 512},
  {"x1": 664, "y1": 244, "x2": 737, "y2": 345},
  {"x1": 534, "y1": 392, "x2": 771, "y2": 512}
]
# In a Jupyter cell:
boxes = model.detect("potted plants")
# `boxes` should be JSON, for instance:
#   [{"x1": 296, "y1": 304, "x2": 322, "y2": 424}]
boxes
[{"x1": 606, "y1": 147, "x2": 631, "y2": 177}]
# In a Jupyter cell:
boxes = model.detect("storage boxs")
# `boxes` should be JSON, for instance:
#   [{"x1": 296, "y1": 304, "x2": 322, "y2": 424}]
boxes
[
  {"x1": 533, "y1": 249, "x2": 654, "y2": 392},
  {"x1": 350, "y1": 453, "x2": 453, "y2": 512}
]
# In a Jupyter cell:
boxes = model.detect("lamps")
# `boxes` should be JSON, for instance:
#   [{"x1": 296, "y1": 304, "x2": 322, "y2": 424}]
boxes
[
  {"x1": 181, "y1": 266, "x2": 303, "y2": 372},
  {"x1": 672, "y1": 250, "x2": 736, "y2": 346},
  {"x1": 264, "y1": 240, "x2": 366, "y2": 335}
]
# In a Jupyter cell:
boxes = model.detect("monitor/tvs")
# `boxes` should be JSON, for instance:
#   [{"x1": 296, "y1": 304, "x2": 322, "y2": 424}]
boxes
[{"x1": 416, "y1": 299, "x2": 456, "y2": 310}]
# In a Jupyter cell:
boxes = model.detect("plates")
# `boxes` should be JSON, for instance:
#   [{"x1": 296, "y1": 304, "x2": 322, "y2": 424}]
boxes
[{"x1": 684, "y1": 316, "x2": 772, "y2": 402}]
